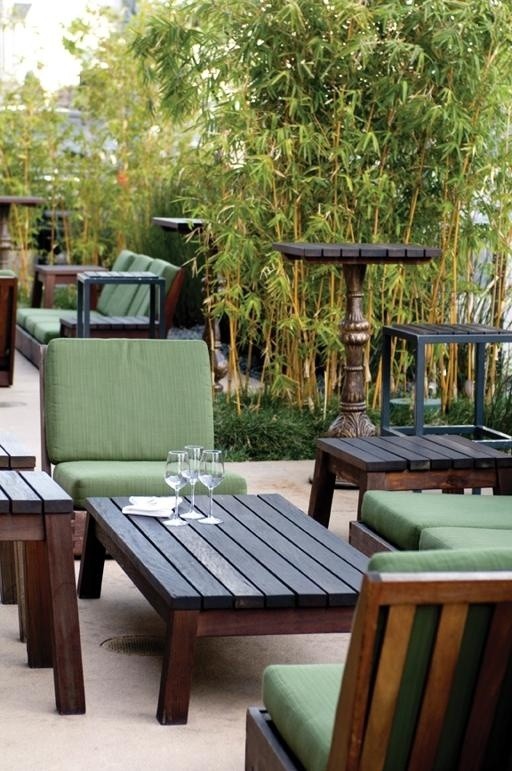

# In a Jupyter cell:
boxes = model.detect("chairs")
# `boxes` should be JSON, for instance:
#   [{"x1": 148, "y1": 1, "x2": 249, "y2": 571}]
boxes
[
  {"x1": 2, "y1": 445, "x2": 86, "y2": 714},
  {"x1": 348, "y1": 491, "x2": 510, "y2": 557},
  {"x1": 38, "y1": 339, "x2": 245, "y2": 556}
]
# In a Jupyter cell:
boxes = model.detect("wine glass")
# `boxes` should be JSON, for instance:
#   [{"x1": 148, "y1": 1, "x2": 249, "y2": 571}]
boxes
[
  {"x1": 180, "y1": 445, "x2": 205, "y2": 520},
  {"x1": 197, "y1": 449, "x2": 225, "y2": 525},
  {"x1": 162, "y1": 450, "x2": 189, "y2": 526}
]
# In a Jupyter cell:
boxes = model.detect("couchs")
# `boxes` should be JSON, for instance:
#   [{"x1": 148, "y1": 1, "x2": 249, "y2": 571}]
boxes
[
  {"x1": 246, "y1": 545, "x2": 511, "y2": 770},
  {"x1": 16, "y1": 251, "x2": 183, "y2": 370}
]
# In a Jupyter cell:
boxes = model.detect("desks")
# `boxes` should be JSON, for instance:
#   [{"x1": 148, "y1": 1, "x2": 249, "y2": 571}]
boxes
[
  {"x1": 35, "y1": 265, "x2": 110, "y2": 310},
  {"x1": 79, "y1": 493, "x2": 372, "y2": 726}
]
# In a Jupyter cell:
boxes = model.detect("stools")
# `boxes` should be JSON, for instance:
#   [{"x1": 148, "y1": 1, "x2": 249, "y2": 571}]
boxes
[{"x1": 305, "y1": 436, "x2": 511, "y2": 528}]
